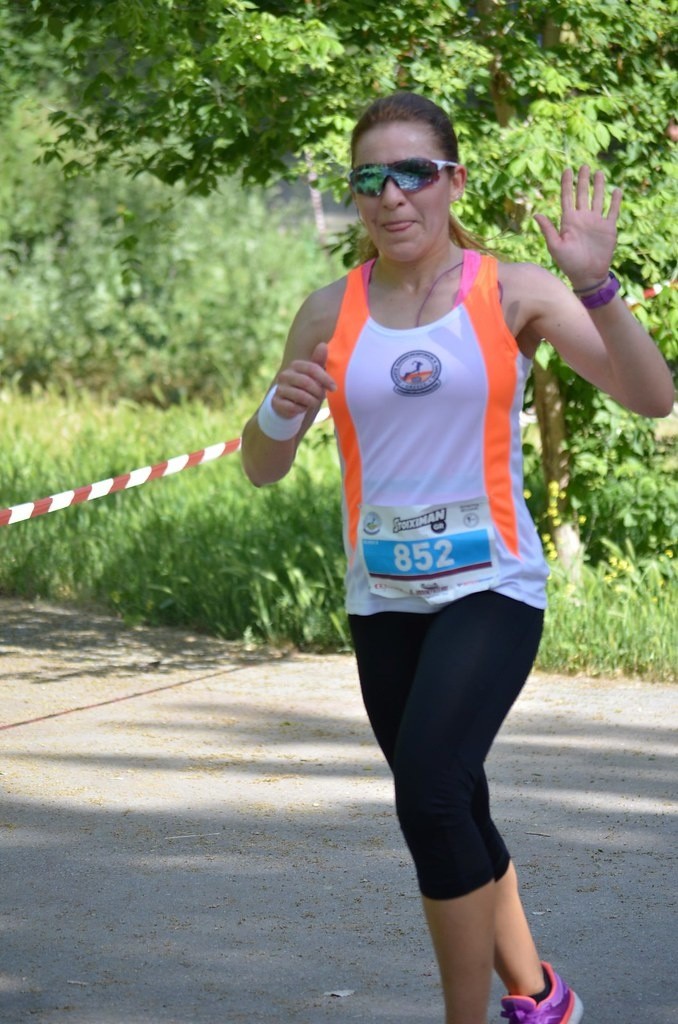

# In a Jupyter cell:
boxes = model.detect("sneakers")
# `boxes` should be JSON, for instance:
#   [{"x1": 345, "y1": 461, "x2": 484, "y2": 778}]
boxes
[{"x1": 501, "y1": 960, "x2": 583, "y2": 1024}]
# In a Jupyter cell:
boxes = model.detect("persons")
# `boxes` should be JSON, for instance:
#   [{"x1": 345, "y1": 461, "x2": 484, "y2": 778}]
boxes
[{"x1": 242, "y1": 93, "x2": 674, "y2": 1024}]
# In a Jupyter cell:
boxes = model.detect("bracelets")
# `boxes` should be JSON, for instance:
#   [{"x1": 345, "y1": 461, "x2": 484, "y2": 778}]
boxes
[
  {"x1": 573, "y1": 271, "x2": 621, "y2": 309},
  {"x1": 258, "y1": 385, "x2": 309, "y2": 441}
]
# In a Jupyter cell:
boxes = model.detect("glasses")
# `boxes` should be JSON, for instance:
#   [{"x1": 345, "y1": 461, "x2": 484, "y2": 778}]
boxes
[{"x1": 349, "y1": 158, "x2": 460, "y2": 198}]
